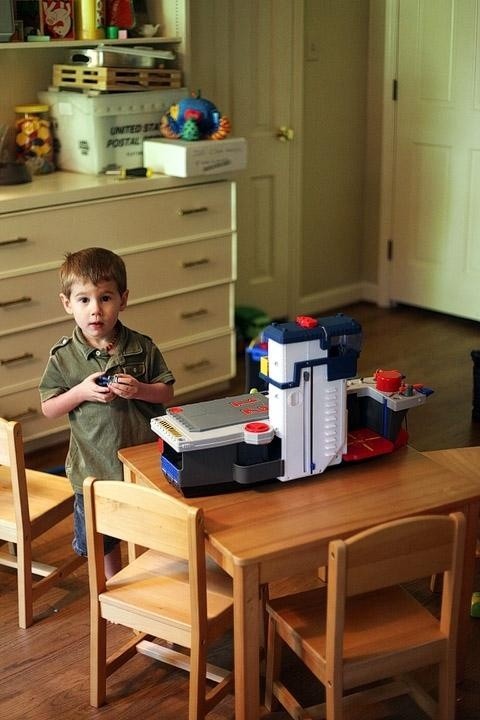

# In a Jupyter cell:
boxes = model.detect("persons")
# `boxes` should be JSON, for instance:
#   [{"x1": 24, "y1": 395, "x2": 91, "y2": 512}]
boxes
[{"x1": 37, "y1": 246, "x2": 177, "y2": 626}]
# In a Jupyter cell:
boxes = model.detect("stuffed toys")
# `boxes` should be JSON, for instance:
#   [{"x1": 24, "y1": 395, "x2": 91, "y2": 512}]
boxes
[{"x1": 158, "y1": 88, "x2": 232, "y2": 141}]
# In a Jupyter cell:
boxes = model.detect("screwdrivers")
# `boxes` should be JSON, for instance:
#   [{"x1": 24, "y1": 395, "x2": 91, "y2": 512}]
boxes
[{"x1": 105, "y1": 167, "x2": 152, "y2": 179}]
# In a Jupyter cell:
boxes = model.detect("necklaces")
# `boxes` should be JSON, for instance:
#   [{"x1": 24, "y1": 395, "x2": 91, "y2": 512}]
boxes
[{"x1": 104, "y1": 326, "x2": 120, "y2": 352}]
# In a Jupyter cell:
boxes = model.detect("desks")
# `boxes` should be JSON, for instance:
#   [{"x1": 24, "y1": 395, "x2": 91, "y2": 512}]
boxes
[{"x1": 114, "y1": 431, "x2": 480, "y2": 720}]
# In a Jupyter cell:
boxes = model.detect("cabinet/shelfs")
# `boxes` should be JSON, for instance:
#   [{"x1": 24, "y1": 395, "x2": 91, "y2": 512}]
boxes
[{"x1": 0, "y1": 0, "x2": 237, "y2": 453}]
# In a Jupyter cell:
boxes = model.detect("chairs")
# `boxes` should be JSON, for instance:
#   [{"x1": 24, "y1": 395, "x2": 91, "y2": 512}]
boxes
[
  {"x1": 0, "y1": 418, "x2": 105, "y2": 630},
  {"x1": 80, "y1": 475, "x2": 269, "y2": 720},
  {"x1": 417, "y1": 444, "x2": 480, "y2": 592},
  {"x1": 262, "y1": 509, "x2": 466, "y2": 720}
]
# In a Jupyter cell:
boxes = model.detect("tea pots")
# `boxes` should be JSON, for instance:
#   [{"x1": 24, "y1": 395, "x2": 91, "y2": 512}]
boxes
[{"x1": 138, "y1": 22, "x2": 161, "y2": 38}]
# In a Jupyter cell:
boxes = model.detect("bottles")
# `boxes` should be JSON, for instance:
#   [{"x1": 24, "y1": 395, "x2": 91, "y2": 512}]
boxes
[{"x1": 12, "y1": 103, "x2": 57, "y2": 177}]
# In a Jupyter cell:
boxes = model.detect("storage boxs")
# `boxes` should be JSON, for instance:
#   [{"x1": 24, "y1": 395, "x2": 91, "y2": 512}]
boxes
[
  {"x1": 37, "y1": 86, "x2": 189, "y2": 174},
  {"x1": 142, "y1": 135, "x2": 248, "y2": 178}
]
